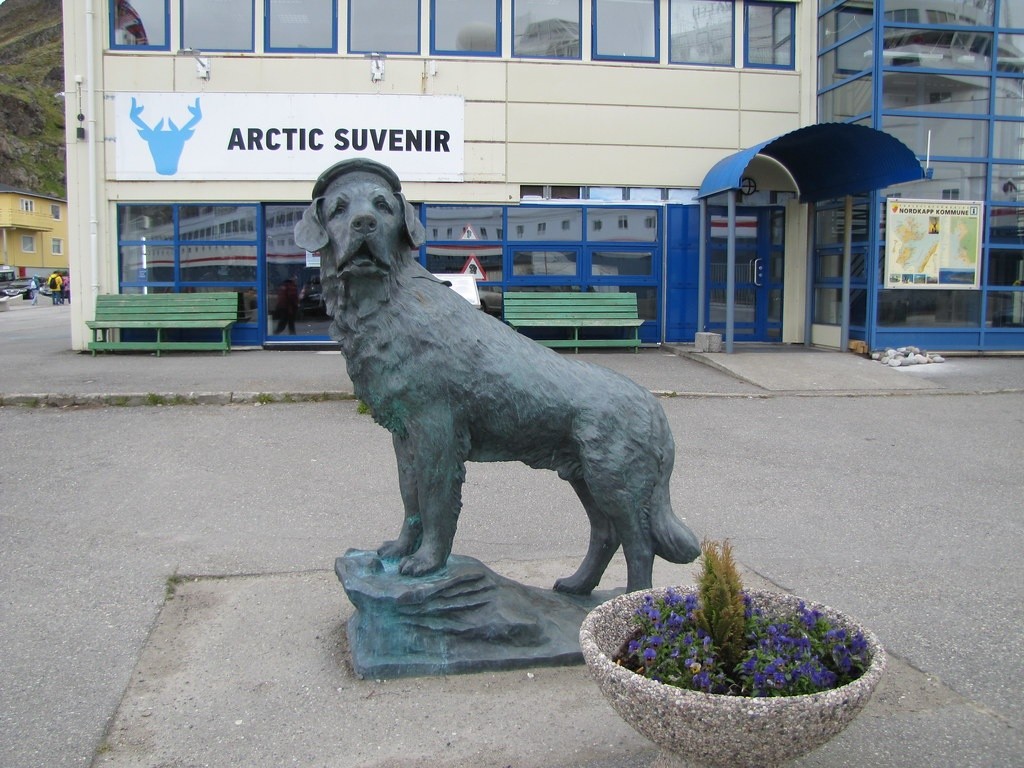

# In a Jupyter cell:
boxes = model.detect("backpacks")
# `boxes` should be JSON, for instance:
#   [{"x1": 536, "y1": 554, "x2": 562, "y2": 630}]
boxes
[
  {"x1": 31, "y1": 280, "x2": 36, "y2": 289},
  {"x1": 50, "y1": 276, "x2": 58, "y2": 289}
]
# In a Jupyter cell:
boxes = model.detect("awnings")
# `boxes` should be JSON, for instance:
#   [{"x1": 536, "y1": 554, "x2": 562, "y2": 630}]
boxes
[{"x1": 693, "y1": 122, "x2": 926, "y2": 355}]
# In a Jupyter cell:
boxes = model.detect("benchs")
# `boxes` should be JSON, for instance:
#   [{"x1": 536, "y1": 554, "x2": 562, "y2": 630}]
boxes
[
  {"x1": 87, "y1": 291, "x2": 239, "y2": 357},
  {"x1": 502, "y1": 293, "x2": 644, "y2": 353}
]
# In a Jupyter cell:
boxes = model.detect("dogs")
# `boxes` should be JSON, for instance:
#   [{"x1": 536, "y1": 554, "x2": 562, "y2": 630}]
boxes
[{"x1": 293, "y1": 157, "x2": 701, "y2": 598}]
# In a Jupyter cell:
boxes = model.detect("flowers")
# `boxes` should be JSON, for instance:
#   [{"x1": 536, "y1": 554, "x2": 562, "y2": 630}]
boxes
[{"x1": 609, "y1": 538, "x2": 876, "y2": 696}]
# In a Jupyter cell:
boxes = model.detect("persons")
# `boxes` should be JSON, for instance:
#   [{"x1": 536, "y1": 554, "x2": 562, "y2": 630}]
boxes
[
  {"x1": 30, "y1": 276, "x2": 40, "y2": 306},
  {"x1": 273, "y1": 280, "x2": 298, "y2": 335},
  {"x1": 46, "y1": 271, "x2": 70, "y2": 305}
]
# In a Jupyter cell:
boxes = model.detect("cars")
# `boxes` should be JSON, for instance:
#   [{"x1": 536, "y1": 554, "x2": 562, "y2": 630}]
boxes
[
  {"x1": 41, "y1": 277, "x2": 68, "y2": 297},
  {"x1": 478, "y1": 286, "x2": 503, "y2": 313},
  {"x1": 3, "y1": 279, "x2": 40, "y2": 299}
]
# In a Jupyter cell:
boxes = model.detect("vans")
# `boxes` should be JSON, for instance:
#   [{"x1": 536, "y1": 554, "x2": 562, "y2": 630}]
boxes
[{"x1": 0, "y1": 270, "x2": 15, "y2": 293}]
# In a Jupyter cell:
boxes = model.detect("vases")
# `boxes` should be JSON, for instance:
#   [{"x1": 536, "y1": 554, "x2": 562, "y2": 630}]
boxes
[{"x1": 581, "y1": 586, "x2": 889, "y2": 768}]
